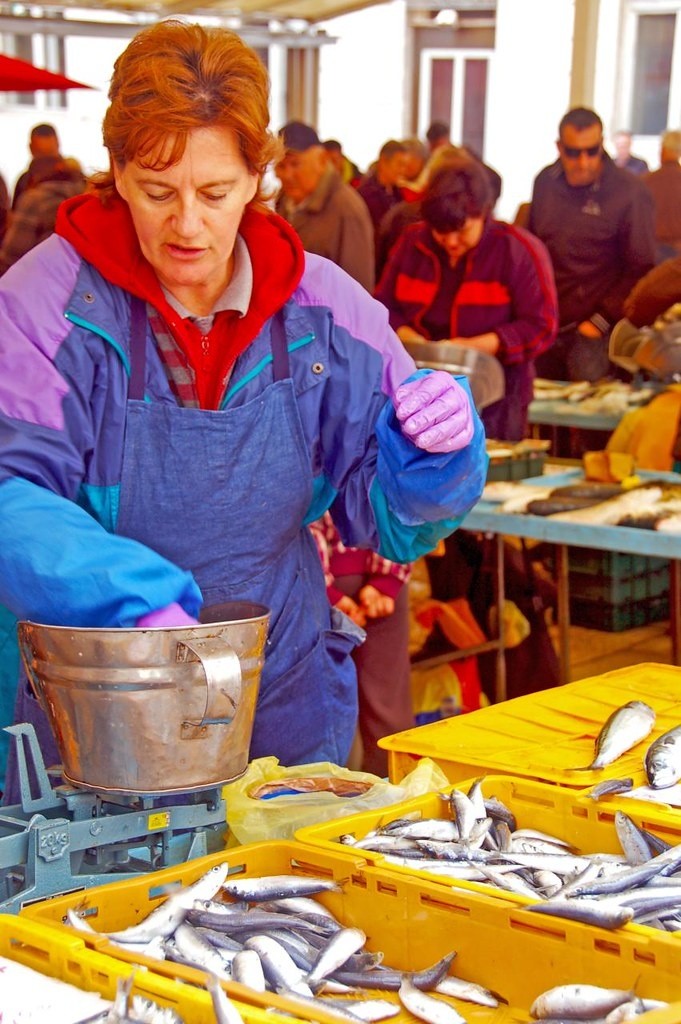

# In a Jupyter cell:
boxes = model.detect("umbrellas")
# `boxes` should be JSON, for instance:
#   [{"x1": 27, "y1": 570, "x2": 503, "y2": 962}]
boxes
[{"x1": 1, "y1": 52, "x2": 97, "y2": 93}]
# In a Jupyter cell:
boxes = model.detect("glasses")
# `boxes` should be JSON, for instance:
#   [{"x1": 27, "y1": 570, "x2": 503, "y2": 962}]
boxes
[{"x1": 563, "y1": 143, "x2": 600, "y2": 158}]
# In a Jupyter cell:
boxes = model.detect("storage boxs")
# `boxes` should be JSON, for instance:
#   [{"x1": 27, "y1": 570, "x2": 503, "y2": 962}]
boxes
[
  {"x1": 486, "y1": 450, "x2": 544, "y2": 482},
  {"x1": 0, "y1": 661, "x2": 681, "y2": 1023},
  {"x1": 542, "y1": 544, "x2": 671, "y2": 632}
]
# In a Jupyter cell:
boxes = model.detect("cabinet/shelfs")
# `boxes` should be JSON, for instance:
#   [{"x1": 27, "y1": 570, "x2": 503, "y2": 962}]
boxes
[{"x1": 410, "y1": 378, "x2": 681, "y2": 704}]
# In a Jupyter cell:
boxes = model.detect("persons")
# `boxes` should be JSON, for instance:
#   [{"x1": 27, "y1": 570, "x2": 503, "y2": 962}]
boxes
[
  {"x1": 2, "y1": 108, "x2": 681, "y2": 710},
  {"x1": 0, "y1": 18, "x2": 487, "y2": 774}
]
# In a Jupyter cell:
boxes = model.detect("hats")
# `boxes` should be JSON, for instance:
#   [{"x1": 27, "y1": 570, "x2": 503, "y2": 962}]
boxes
[{"x1": 279, "y1": 121, "x2": 320, "y2": 151}]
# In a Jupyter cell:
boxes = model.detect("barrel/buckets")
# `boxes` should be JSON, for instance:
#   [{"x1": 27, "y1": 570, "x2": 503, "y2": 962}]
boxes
[
  {"x1": 400, "y1": 335, "x2": 506, "y2": 416},
  {"x1": 15, "y1": 601, "x2": 273, "y2": 798}
]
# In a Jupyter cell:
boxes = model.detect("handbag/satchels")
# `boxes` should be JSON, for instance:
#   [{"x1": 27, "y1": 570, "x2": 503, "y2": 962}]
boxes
[
  {"x1": 608, "y1": 299, "x2": 680, "y2": 377},
  {"x1": 220, "y1": 755, "x2": 450, "y2": 851}
]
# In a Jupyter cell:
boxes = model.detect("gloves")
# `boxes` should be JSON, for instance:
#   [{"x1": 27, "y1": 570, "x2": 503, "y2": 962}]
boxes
[
  {"x1": 392, "y1": 370, "x2": 474, "y2": 453},
  {"x1": 135, "y1": 602, "x2": 201, "y2": 628}
]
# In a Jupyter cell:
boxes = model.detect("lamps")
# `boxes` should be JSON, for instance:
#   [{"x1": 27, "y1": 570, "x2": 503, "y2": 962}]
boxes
[{"x1": 435, "y1": 9, "x2": 459, "y2": 29}]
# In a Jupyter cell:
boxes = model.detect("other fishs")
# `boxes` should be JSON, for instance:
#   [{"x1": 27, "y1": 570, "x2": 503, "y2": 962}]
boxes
[{"x1": 61, "y1": 698, "x2": 681, "y2": 1024}]
